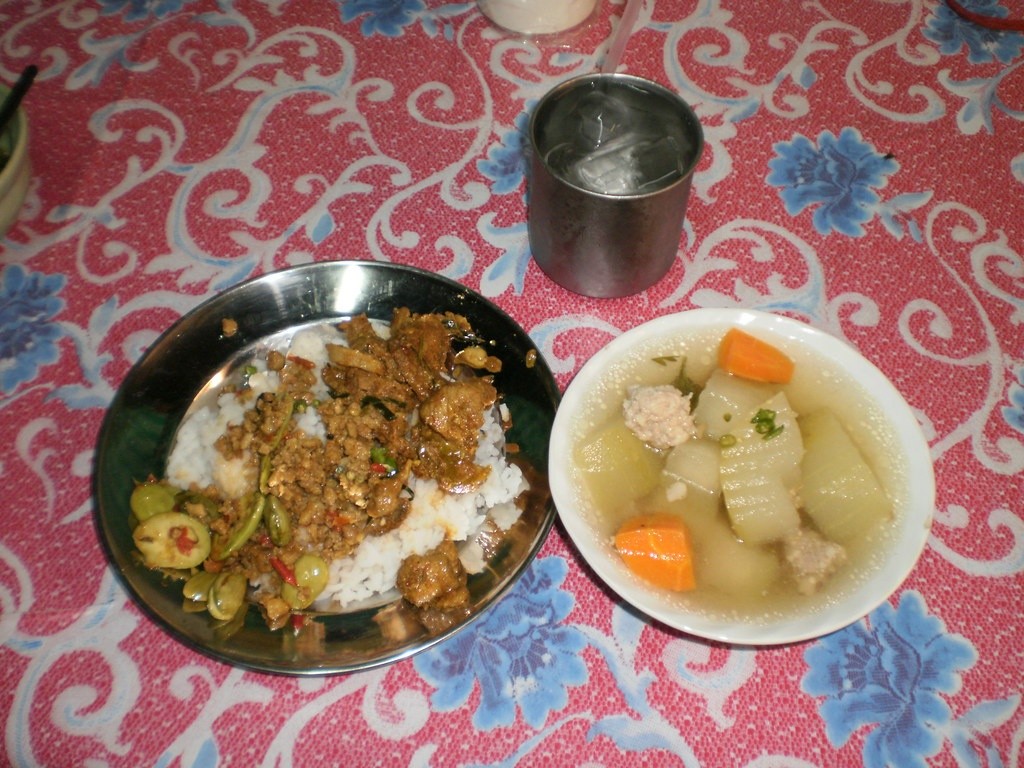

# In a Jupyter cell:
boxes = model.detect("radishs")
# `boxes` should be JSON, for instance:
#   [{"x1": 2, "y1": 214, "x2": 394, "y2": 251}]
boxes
[{"x1": 579, "y1": 354, "x2": 890, "y2": 598}]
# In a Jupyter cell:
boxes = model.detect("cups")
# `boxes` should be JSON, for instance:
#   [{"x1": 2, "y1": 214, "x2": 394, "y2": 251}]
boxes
[
  {"x1": 525, "y1": 73, "x2": 703, "y2": 299},
  {"x1": 478, "y1": 1, "x2": 597, "y2": 38},
  {"x1": 0, "y1": 83, "x2": 30, "y2": 237}
]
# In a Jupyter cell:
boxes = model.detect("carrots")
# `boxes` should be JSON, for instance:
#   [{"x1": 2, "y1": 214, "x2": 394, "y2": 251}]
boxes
[
  {"x1": 614, "y1": 514, "x2": 696, "y2": 594},
  {"x1": 715, "y1": 328, "x2": 796, "y2": 383}
]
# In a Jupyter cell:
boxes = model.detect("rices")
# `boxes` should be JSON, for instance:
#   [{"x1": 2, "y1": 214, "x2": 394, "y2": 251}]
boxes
[{"x1": 164, "y1": 328, "x2": 527, "y2": 607}]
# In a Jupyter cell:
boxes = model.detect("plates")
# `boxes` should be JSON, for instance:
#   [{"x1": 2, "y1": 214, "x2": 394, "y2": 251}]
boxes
[
  {"x1": 96, "y1": 259, "x2": 560, "y2": 676},
  {"x1": 548, "y1": 308, "x2": 935, "y2": 646}
]
